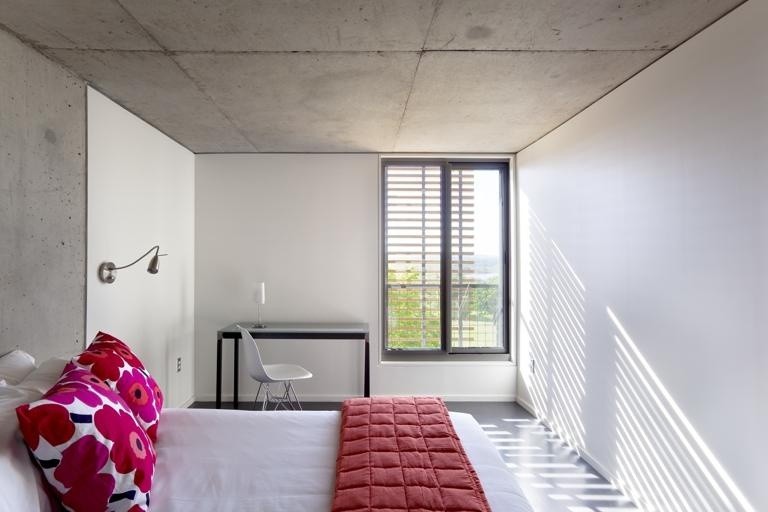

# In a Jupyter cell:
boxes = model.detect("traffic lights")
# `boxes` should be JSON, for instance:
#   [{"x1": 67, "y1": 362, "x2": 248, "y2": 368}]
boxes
[
  {"x1": 72, "y1": 329, "x2": 162, "y2": 443},
  {"x1": 18, "y1": 361, "x2": 157, "y2": 512}
]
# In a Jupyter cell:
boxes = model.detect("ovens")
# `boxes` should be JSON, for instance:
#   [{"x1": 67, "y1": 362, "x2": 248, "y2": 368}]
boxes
[{"x1": 1, "y1": 350, "x2": 533, "y2": 512}]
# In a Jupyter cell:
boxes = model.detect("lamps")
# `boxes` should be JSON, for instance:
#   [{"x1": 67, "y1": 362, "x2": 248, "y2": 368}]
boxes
[{"x1": 236, "y1": 324, "x2": 312, "y2": 410}]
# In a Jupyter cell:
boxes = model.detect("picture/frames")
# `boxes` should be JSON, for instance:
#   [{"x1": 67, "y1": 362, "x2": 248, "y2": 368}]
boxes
[{"x1": 216, "y1": 321, "x2": 369, "y2": 408}]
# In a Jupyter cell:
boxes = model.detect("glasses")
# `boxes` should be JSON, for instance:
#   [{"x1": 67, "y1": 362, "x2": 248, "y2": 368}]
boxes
[
  {"x1": 252, "y1": 281, "x2": 265, "y2": 327},
  {"x1": 99, "y1": 245, "x2": 159, "y2": 283}
]
